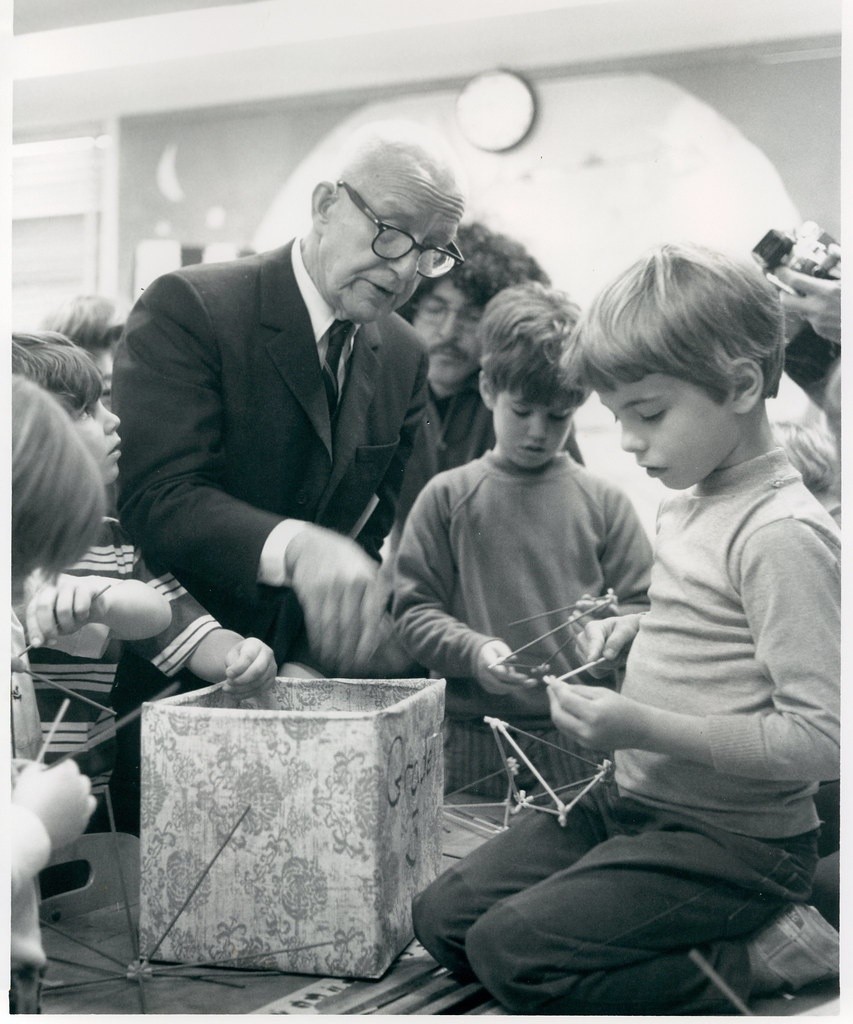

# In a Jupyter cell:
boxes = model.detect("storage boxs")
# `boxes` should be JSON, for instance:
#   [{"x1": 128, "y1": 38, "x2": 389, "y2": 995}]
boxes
[{"x1": 137, "y1": 676, "x2": 447, "y2": 980}]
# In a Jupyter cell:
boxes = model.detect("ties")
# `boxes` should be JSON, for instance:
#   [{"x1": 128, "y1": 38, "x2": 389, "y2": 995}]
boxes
[{"x1": 318, "y1": 319, "x2": 355, "y2": 422}]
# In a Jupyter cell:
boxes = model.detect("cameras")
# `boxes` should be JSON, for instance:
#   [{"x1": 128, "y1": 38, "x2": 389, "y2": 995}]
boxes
[{"x1": 751, "y1": 221, "x2": 840, "y2": 299}]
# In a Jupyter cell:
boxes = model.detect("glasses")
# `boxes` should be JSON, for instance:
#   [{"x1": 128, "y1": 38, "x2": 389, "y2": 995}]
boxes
[{"x1": 334, "y1": 172, "x2": 467, "y2": 279}]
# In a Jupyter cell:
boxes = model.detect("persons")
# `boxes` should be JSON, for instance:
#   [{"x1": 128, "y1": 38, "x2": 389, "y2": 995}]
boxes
[
  {"x1": 392, "y1": 283, "x2": 655, "y2": 805},
  {"x1": 11, "y1": 142, "x2": 841, "y2": 1014},
  {"x1": 412, "y1": 242, "x2": 841, "y2": 1016}
]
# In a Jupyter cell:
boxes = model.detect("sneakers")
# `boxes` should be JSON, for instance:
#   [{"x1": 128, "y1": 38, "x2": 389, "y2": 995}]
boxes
[{"x1": 754, "y1": 899, "x2": 843, "y2": 990}]
